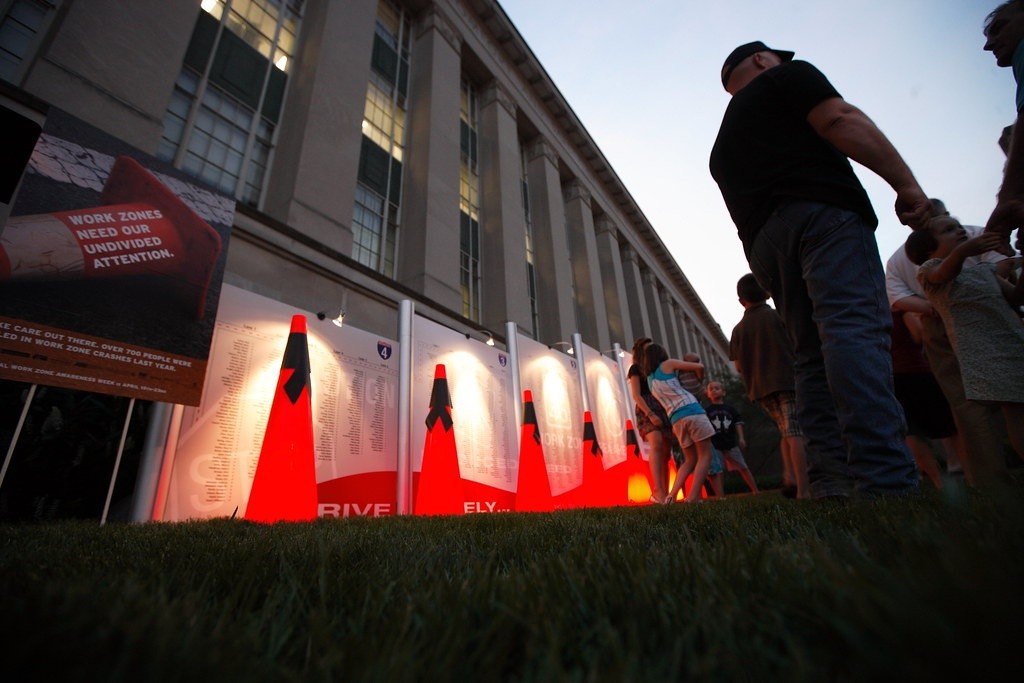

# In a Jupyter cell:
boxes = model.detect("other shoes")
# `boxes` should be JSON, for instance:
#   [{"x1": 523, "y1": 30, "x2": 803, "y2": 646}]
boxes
[{"x1": 650, "y1": 494, "x2": 674, "y2": 506}]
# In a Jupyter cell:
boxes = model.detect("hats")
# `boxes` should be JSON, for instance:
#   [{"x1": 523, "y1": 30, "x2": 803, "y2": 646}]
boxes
[{"x1": 722, "y1": 41, "x2": 794, "y2": 86}]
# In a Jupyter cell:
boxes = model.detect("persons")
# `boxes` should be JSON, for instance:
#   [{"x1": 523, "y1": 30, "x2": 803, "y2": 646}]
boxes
[
  {"x1": 708, "y1": 0, "x2": 1023, "y2": 504},
  {"x1": 628, "y1": 335, "x2": 764, "y2": 503}
]
